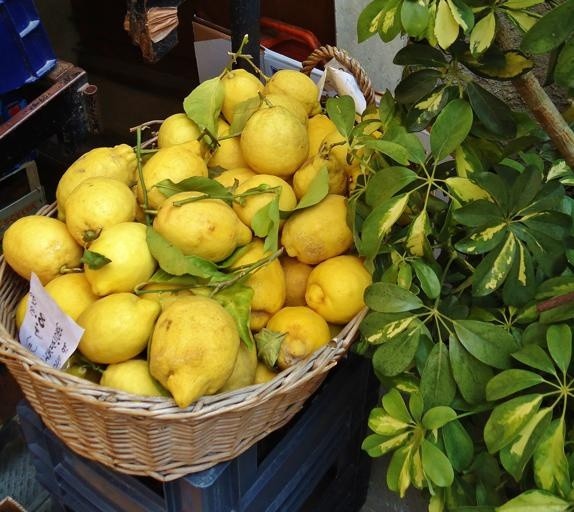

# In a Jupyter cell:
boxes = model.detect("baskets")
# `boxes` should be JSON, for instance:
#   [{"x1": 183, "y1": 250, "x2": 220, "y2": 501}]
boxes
[{"x1": 0, "y1": 43, "x2": 385, "y2": 483}]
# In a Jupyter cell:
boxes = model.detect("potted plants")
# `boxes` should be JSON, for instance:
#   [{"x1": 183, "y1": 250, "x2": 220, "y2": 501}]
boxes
[{"x1": 326, "y1": 0, "x2": 574, "y2": 512}]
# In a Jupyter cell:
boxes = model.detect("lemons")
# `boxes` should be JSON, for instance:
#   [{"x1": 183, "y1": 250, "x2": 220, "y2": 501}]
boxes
[{"x1": 2, "y1": 69, "x2": 423, "y2": 407}]
